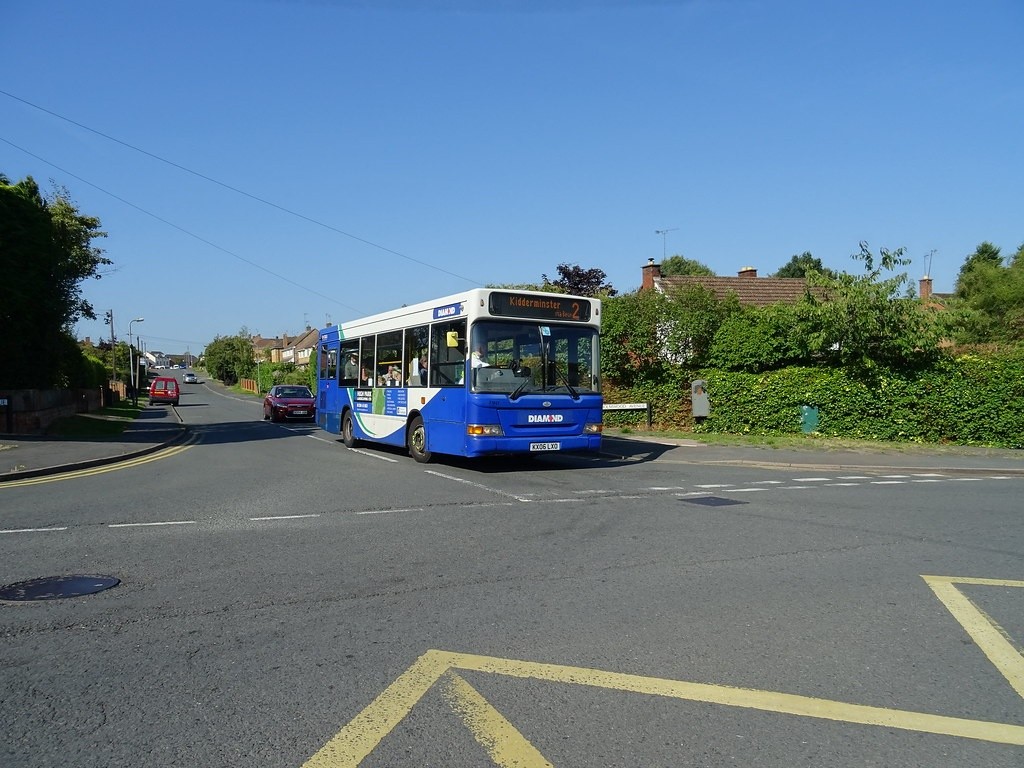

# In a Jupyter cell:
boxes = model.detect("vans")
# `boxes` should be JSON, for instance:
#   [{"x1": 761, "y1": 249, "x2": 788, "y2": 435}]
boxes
[{"x1": 147, "y1": 377, "x2": 181, "y2": 406}]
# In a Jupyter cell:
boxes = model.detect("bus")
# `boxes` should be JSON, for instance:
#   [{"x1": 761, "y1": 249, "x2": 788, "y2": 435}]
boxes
[{"x1": 314, "y1": 288, "x2": 605, "y2": 465}]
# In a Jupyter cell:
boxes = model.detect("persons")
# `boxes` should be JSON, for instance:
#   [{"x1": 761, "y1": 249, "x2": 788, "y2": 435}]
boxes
[
  {"x1": 384, "y1": 365, "x2": 400, "y2": 382},
  {"x1": 277, "y1": 389, "x2": 284, "y2": 397},
  {"x1": 419, "y1": 357, "x2": 428, "y2": 382},
  {"x1": 459, "y1": 342, "x2": 491, "y2": 384},
  {"x1": 345, "y1": 353, "x2": 367, "y2": 381}
]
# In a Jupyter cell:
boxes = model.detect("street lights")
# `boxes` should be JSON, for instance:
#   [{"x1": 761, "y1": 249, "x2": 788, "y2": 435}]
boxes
[{"x1": 128, "y1": 317, "x2": 145, "y2": 406}]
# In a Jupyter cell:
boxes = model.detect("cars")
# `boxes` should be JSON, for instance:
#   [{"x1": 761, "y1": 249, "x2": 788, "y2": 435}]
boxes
[
  {"x1": 182, "y1": 373, "x2": 198, "y2": 384},
  {"x1": 261, "y1": 385, "x2": 316, "y2": 423}
]
